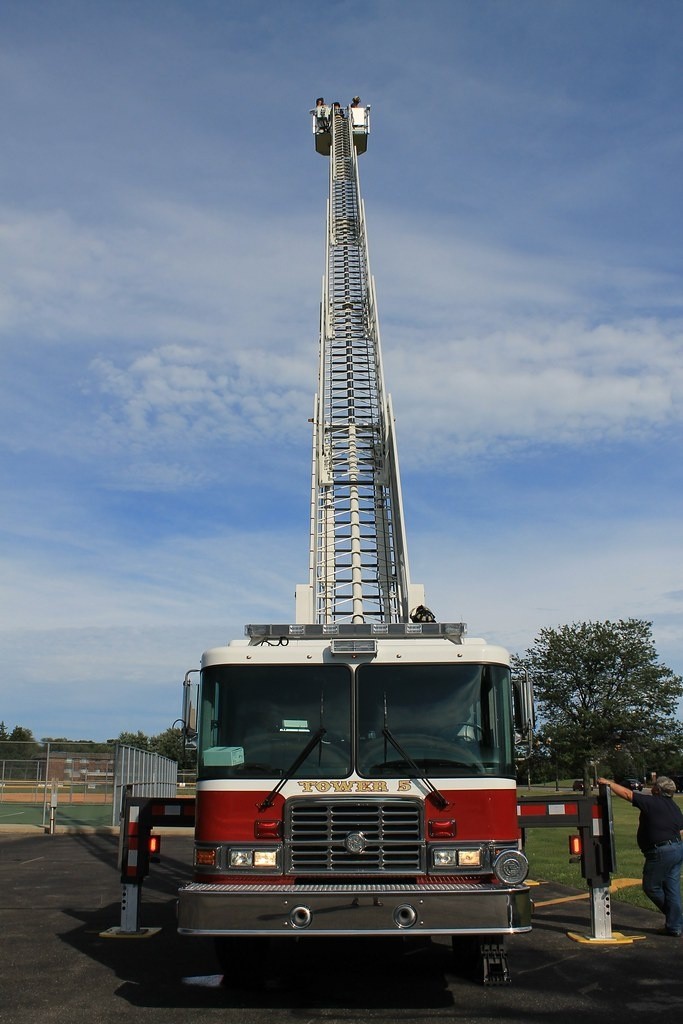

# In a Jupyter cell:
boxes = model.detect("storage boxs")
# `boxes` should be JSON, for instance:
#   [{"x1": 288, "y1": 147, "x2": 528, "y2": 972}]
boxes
[{"x1": 202, "y1": 746, "x2": 244, "y2": 766}]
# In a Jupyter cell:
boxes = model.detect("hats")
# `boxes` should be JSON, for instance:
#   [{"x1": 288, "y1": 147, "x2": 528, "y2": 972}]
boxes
[{"x1": 655, "y1": 776, "x2": 677, "y2": 791}]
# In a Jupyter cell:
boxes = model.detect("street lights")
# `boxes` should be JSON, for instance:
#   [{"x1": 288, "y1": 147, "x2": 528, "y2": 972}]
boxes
[
  {"x1": 536, "y1": 740, "x2": 546, "y2": 786},
  {"x1": 547, "y1": 737, "x2": 560, "y2": 791}
]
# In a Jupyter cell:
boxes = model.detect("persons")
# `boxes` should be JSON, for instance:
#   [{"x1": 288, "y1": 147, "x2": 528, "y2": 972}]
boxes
[{"x1": 597, "y1": 776, "x2": 683, "y2": 937}]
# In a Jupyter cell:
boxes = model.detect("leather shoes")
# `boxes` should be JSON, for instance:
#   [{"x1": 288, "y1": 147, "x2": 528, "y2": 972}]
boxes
[{"x1": 656, "y1": 927, "x2": 682, "y2": 937}]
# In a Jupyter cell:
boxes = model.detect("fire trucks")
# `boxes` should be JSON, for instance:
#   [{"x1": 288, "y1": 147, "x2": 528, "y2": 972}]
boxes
[{"x1": 95, "y1": 94, "x2": 648, "y2": 998}]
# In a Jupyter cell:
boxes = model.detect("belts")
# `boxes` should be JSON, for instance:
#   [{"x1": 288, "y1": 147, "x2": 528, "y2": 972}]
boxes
[{"x1": 640, "y1": 838, "x2": 682, "y2": 851}]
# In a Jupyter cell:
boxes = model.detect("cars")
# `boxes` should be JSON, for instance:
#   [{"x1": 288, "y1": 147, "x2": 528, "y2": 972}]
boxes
[
  {"x1": 620, "y1": 779, "x2": 645, "y2": 791},
  {"x1": 668, "y1": 775, "x2": 683, "y2": 793},
  {"x1": 572, "y1": 779, "x2": 593, "y2": 791}
]
